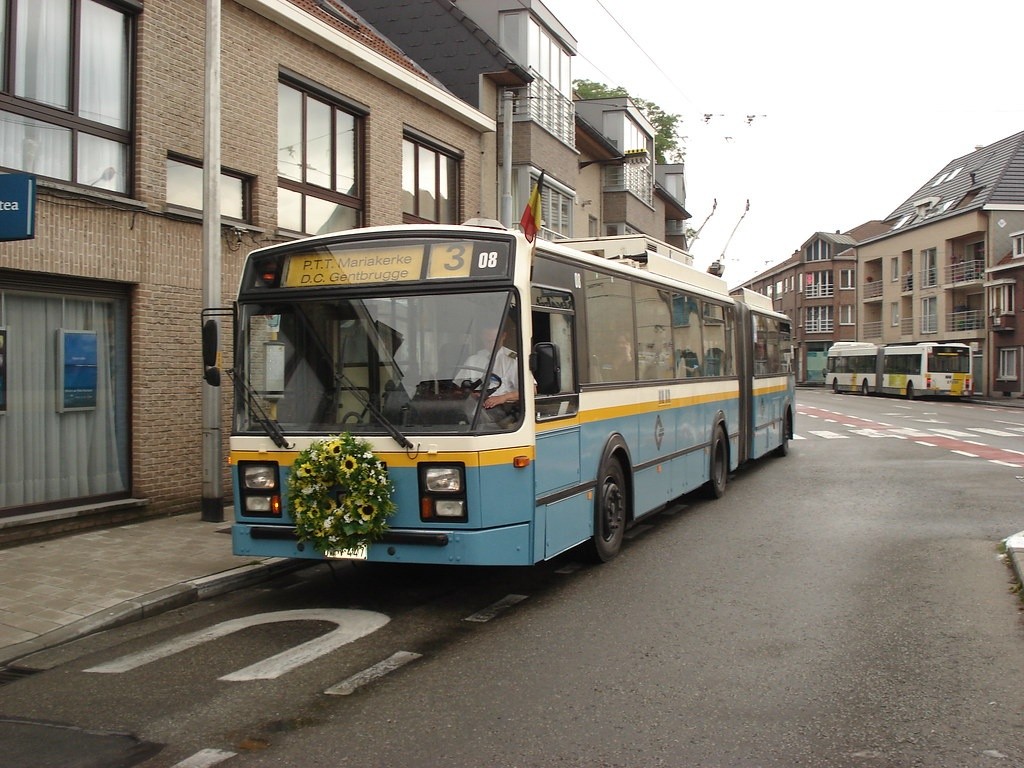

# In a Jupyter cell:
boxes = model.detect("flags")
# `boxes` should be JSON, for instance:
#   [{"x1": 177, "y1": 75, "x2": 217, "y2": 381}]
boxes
[{"x1": 520, "y1": 169, "x2": 544, "y2": 243}]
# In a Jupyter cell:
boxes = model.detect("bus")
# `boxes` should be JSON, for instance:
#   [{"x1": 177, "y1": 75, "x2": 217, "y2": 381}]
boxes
[
  {"x1": 823, "y1": 341, "x2": 974, "y2": 401},
  {"x1": 200, "y1": 198, "x2": 795, "y2": 566}
]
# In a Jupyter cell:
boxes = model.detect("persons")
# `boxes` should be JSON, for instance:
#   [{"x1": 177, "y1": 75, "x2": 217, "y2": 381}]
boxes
[
  {"x1": 757, "y1": 333, "x2": 771, "y2": 374},
  {"x1": 578, "y1": 331, "x2": 697, "y2": 382},
  {"x1": 452, "y1": 317, "x2": 537, "y2": 409}
]
